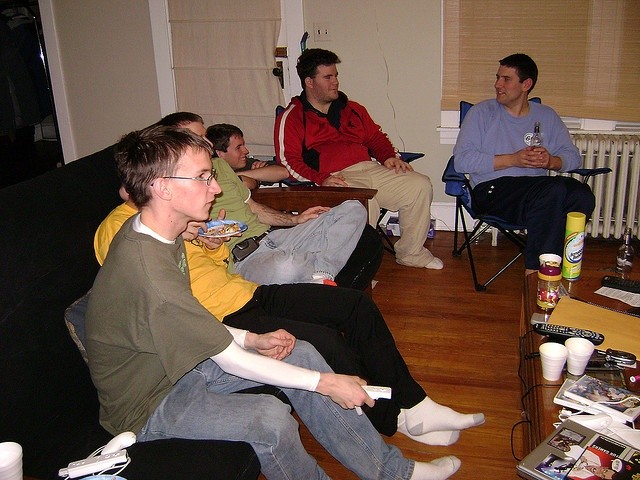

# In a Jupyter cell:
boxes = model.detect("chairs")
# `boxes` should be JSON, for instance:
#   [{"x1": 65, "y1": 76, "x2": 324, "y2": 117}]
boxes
[
  {"x1": 443, "y1": 99, "x2": 611, "y2": 291},
  {"x1": 274, "y1": 105, "x2": 424, "y2": 257}
]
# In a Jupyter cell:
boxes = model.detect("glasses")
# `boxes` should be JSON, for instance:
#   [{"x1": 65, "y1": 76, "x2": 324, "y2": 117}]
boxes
[{"x1": 150, "y1": 169, "x2": 216, "y2": 186}]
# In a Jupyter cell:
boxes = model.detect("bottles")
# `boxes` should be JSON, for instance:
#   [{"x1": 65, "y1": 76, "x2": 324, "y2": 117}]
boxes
[
  {"x1": 531, "y1": 122, "x2": 542, "y2": 147},
  {"x1": 538, "y1": 263, "x2": 561, "y2": 313},
  {"x1": 617, "y1": 227, "x2": 633, "y2": 280},
  {"x1": 562, "y1": 211, "x2": 585, "y2": 282}
]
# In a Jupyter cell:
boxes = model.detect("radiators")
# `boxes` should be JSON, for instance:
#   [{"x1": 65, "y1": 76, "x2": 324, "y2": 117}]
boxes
[{"x1": 492, "y1": 132, "x2": 639, "y2": 248}]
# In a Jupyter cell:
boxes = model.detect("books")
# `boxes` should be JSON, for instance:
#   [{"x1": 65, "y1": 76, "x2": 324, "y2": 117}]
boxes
[
  {"x1": 564, "y1": 374, "x2": 640, "y2": 422},
  {"x1": 516, "y1": 419, "x2": 640, "y2": 480},
  {"x1": 553, "y1": 378, "x2": 627, "y2": 424}
]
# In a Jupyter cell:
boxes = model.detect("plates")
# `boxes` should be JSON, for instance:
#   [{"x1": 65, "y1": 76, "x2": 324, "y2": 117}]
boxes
[{"x1": 196, "y1": 220, "x2": 248, "y2": 238}]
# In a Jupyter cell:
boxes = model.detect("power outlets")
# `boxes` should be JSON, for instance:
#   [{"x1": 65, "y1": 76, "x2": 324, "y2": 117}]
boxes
[{"x1": 313, "y1": 22, "x2": 333, "y2": 43}]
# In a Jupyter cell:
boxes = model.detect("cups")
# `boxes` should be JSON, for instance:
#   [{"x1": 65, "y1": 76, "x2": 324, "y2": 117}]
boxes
[
  {"x1": 538, "y1": 254, "x2": 561, "y2": 266},
  {"x1": 539, "y1": 341, "x2": 568, "y2": 382},
  {"x1": 565, "y1": 339, "x2": 594, "y2": 376}
]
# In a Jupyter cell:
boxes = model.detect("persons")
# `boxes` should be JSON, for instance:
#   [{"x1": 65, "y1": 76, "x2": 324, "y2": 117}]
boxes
[
  {"x1": 93, "y1": 193, "x2": 486, "y2": 448},
  {"x1": 452, "y1": 53, "x2": 596, "y2": 278},
  {"x1": 206, "y1": 123, "x2": 290, "y2": 190},
  {"x1": 567, "y1": 449, "x2": 617, "y2": 480},
  {"x1": 550, "y1": 436, "x2": 584, "y2": 461},
  {"x1": 273, "y1": 47, "x2": 445, "y2": 271},
  {"x1": 84, "y1": 125, "x2": 462, "y2": 480},
  {"x1": 160, "y1": 111, "x2": 368, "y2": 287}
]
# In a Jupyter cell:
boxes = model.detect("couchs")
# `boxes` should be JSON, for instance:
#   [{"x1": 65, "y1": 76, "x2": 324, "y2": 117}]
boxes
[{"x1": 0, "y1": 143, "x2": 377, "y2": 477}]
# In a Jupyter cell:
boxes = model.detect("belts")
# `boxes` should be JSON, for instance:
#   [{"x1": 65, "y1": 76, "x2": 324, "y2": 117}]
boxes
[{"x1": 233, "y1": 228, "x2": 274, "y2": 263}]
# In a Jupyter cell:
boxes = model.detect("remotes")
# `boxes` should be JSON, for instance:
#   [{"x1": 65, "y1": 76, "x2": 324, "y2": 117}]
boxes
[
  {"x1": 356, "y1": 385, "x2": 394, "y2": 403},
  {"x1": 602, "y1": 274, "x2": 640, "y2": 293},
  {"x1": 100, "y1": 431, "x2": 138, "y2": 456},
  {"x1": 533, "y1": 321, "x2": 605, "y2": 347},
  {"x1": 66, "y1": 448, "x2": 127, "y2": 479},
  {"x1": 566, "y1": 410, "x2": 614, "y2": 432}
]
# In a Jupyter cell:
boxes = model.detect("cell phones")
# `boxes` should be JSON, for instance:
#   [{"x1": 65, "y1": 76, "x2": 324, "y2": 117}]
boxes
[{"x1": 596, "y1": 348, "x2": 636, "y2": 365}]
